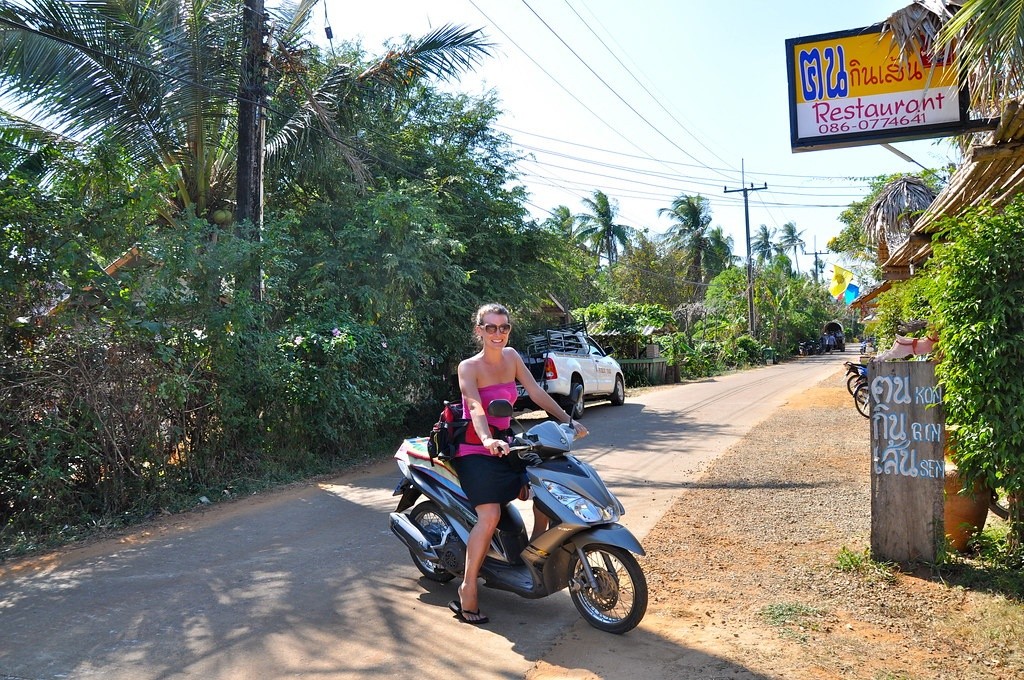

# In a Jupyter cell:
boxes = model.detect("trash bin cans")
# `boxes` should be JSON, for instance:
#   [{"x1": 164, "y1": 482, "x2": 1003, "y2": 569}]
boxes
[{"x1": 762, "y1": 348, "x2": 775, "y2": 365}]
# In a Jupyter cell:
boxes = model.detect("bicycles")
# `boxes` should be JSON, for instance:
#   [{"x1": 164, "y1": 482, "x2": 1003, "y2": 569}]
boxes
[{"x1": 853, "y1": 374, "x2": 870, "y2": 419}]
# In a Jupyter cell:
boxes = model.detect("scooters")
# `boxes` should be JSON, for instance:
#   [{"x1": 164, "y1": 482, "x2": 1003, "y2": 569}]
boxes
[
  {"x1": 389, "y1": 384, "x2": 649, "y2": 636},
  {"x1": 843, "y1": 362, "x2": 868, "y2": 396},
  {"x1": 798, "y1": 339, "x2": 825, "y2": 356}
]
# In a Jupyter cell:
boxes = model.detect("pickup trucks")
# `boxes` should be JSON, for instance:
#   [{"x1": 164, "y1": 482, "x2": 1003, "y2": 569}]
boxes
[{"x1": 513, "y1": 330, "x2": 626, "y2": 420}]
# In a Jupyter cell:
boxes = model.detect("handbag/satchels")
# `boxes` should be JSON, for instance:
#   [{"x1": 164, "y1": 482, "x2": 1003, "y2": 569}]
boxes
[{"x1": 427, "y1": 403, "x2": 466, "y2": 468}]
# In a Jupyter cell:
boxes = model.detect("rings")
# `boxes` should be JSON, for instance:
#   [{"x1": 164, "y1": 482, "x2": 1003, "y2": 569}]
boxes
[{"x1": 494, "y1": 447, "x2": 497, "y2": 451}]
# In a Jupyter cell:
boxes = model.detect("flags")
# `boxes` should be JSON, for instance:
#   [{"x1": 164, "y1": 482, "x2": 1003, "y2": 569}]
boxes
[
  {"x1": 827, "y1": 266, "x2": 853, "y2": 297},
  {"x1": 845, "y1": 284, "x2": 859, "y2": 305}
]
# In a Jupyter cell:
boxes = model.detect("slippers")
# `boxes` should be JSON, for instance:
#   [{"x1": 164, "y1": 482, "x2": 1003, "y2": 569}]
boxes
[{"x1": 448, "y1": 601, "x2": 489, "y2": 624}]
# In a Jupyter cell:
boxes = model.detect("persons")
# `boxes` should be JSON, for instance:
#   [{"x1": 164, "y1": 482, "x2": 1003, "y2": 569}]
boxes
[
  {"x1": 829, "y1": 329, "x2": 841, "y2": 335},
  {"x1": 819, "y1": 332, "x2": 829, "y2": 355},
  {"x1": 828, "y1": 333, "x2": 837, "y2": 355},
  {"x1": 446, "y1": 303, "x2": 587, "y2": 626}
]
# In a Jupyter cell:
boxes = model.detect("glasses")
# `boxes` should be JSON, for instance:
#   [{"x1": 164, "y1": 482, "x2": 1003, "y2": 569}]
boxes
[{"x1": 480, "y1": 323, "x2": 511, "y2": 334}]
